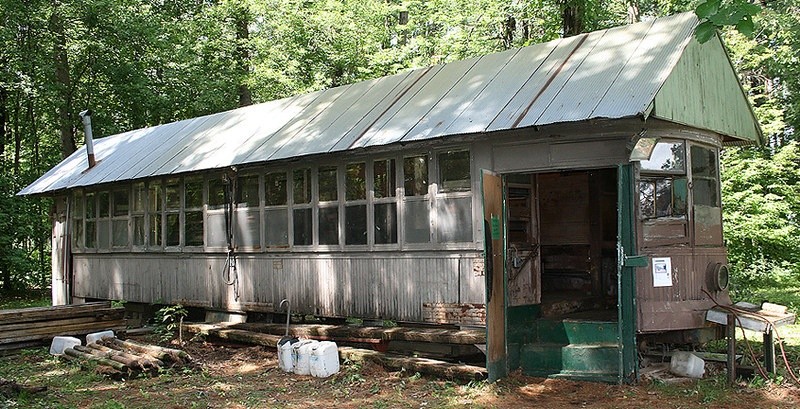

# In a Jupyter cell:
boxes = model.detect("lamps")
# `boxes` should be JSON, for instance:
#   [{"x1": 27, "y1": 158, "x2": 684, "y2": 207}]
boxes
[{"x1": 231, "y1": 165, "x2": 239, "y2": 172}]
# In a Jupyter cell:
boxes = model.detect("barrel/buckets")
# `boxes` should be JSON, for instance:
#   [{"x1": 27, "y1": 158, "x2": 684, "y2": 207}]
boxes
[
  {"x1": 87, "y1": 330, "x2": 117, "y2": 345},
  {"x1": 49, "y1": 336, "x2": 82, "y2": 356},
  {"x1": 662, "y1": 351, "x2": 705, "y2": 378},
  {"x1": 277, "y1": 338, "x2": 340, "y2": 378}
]
